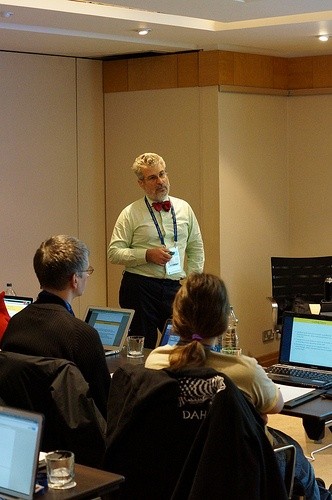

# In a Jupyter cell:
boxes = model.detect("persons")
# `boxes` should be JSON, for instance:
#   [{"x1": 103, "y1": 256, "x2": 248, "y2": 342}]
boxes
[
  {"x1": 108, "y1": 152, "x2": 205, "y2": 350},
  {"x1": 0, "y1": 235, "x2": 111, "y2": 421},
  {"x1": 147, "y1": 274, "x2": 332, "y2": 500}
]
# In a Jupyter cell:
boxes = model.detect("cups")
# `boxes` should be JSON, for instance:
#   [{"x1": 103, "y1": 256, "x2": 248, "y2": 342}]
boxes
[
  {"x1": 221, "y1": 347, "x2": 242, "y2": 355},
  {"x1": 45, "y1": 450, "x2": 74, "y2": 489},
  {"x1": 127, "y1": 336, "x2": 145, "y2": 358}
]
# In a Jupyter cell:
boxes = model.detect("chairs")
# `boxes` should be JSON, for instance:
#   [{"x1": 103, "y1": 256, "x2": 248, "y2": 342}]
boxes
[{"x1": 0, "y1": 349, "x2": 296, "y2": 500}]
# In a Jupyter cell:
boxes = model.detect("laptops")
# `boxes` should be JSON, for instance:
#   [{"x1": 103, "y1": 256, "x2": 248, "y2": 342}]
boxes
[
  {"x1": 158, "y1": 318, "x2": 181, "y2": 347},
  {"x1": 263, "y1": 310, "x2": 332, "y2": 390},
  {"x1": 0, "y1": 405, "x2": 44, "y2": 500},
  {"x1": 83, "y1": 305, "x2": 135, "y2": 357},
  {"x1": 3, "y1": 295, "x2": 33, "y2": 318}
]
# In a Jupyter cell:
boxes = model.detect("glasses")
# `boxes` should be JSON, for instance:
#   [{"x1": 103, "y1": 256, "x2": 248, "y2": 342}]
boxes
[{"x1": 70, "y1": 264, "x2": 94, "y2": 276}]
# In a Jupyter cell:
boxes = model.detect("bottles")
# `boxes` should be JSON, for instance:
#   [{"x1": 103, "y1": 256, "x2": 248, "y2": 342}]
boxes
[
  {"x1": 3, "y1": 283, "x2": 16, "y2": 296},
  {"x1": 223, "y1": 304, "x2": 238, "y2": 348}
]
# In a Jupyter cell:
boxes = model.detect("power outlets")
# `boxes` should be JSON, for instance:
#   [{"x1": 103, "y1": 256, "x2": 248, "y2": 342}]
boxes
[{"x1": 262, "y1": 330, "x2": 274, "y2": 342}]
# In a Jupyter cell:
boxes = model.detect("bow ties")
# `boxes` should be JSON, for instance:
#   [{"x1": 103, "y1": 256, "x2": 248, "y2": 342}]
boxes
[{"x1": 151, "y1": 200, "x2": 171, "y2": 212}]
